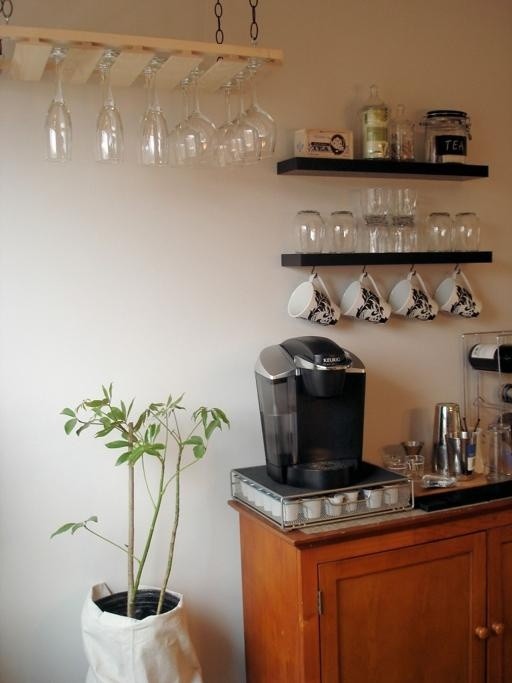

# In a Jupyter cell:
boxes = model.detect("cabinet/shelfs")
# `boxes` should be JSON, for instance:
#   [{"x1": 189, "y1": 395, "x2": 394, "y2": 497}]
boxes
[
  {"x1": 241, "y1": 505, "x2": 512, "y2": 683},
  {"x1": 278, "y1": 156, "x2": 493, "y2": 267}
]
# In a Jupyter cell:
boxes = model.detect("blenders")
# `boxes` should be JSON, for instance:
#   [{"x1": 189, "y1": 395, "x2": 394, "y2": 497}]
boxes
[{"x1": 255, "y1": 335, "x2": 366, "y2": 488}]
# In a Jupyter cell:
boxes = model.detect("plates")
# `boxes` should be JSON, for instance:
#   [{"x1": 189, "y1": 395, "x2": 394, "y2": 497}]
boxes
[{"x1": 423, "y1": 474, "x2": 455, "y2": 486}]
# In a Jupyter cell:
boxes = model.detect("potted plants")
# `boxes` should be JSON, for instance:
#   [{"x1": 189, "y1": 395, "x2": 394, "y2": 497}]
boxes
[{"x1": 47, "y1": 382, "x2": 231, "y2": 683}]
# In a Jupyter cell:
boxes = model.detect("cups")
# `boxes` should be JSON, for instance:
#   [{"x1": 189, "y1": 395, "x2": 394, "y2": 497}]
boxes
[
  {"x1": 294, "y1": 188, "x2": 482, "y2": 253},
  {"x1": 489, "y1": 423, "x2": 511, "y2": 475},
  {"x1": 287, "y1": 270, "x2": 481, "y2": 324},
  {"x1": 385, "y1": 455, "x2": 424, "y2": 478},
  {"x1": 401, "y1": 439, "x2": 424, "y2": 456},
  {"x1": 431, "y1": 402, "x2": 476, "y2": 480},
  {"x1": 239, "y1": 483, "x2": 400, "y2": 523}
]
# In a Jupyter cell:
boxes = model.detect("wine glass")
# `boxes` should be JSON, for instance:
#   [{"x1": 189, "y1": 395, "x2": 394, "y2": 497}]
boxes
[
  {"x1": 46, "y1": 47, "x2": 73, "y2": 160},
  {"x1": 141, "y1": 55, "x2": 277, "y2": 167},
  {"x1": 94, "y1": 51, "x2": 125, "y2": 162}
]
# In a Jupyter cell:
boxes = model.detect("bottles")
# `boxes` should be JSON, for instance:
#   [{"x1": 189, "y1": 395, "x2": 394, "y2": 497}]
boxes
[
  {"x1": 358, "y1": 85, "x2": 390, "y2": 157},
  {"x1": 468, "y1": 342, "x2": 511, "y2": 371},
  {"x1": 389, "y1": 103, "x2": 415, "y2": 159}
]
